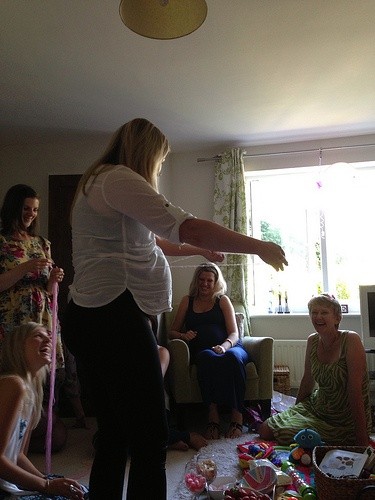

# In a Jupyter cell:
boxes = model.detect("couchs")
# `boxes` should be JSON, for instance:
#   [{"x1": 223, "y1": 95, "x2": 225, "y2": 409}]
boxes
[{"x1": 164, "y1": 298, "x2": 274, "y2": 429}]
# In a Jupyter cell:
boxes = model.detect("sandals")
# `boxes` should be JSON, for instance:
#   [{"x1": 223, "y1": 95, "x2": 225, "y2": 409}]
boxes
[
  {"x1": 206, "y1": 422, "x2": 221, "y2": 440},
  {"x1": 224, "y1": 422, "x2": 243, "y2": 440}
]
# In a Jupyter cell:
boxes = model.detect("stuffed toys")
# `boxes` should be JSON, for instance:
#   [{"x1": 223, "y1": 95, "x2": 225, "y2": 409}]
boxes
[{"x1": 288, "y1": 427, "x2": 326, "y2": 465}]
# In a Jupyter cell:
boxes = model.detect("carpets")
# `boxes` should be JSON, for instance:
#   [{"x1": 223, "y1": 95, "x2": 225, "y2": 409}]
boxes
[{"x1": 174, "y1": 435, "x2": 375, "y2": 500}]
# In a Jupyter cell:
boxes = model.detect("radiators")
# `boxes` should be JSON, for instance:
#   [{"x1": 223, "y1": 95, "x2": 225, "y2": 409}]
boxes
[{"x1": 273, "y1": 339, "x2": 309, "y2": 388}]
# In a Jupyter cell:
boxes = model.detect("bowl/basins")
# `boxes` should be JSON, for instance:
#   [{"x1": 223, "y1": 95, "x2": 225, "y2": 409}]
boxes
[
  {"x1": 185, "y1": 460, "x2": 216, "y2": 495},
  {"x1": 205, "y1": 476, "x2": 237, "y2": 500},
  {"x1": 241, "y1": 465, "x2": 277, "y2": 493}
]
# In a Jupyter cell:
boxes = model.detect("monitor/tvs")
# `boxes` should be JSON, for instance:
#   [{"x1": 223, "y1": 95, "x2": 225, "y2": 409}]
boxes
[{"x1": 359, "y1": 285, "x2": 375, "y2": 352}]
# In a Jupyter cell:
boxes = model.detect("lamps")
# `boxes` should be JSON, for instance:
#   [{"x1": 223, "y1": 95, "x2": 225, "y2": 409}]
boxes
[{"x1": 118, "y1": 0, "x2": 209, "y2": 40}]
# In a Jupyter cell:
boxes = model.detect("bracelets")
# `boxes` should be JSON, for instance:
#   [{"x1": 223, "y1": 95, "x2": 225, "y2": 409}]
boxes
[
  {"x1": 227, "y1": 339, "x2": 233, "y2": 347},
  {"x1": 47, "y1": 479, "x2": 49, "y2": 488}
]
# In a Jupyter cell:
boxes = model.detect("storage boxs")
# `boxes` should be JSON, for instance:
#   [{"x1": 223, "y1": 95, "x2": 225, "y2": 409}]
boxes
[{"x1": 274, "y1": 366, "x2": 291, "y2": 394}]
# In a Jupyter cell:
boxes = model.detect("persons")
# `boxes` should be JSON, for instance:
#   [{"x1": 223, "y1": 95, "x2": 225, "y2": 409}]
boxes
[
  {"x1": 0, "y1": 184, "x2": 65, "y2": 455},
  {"x1": 0, "y1": 321, "x2": 88, "y2": 500},
  {"x1": 259, "y1": 297, "x2": 375, "y2": 447},
  {"x1": 169, "y1": 263, "x2": 249, "y2": 439},
  {"x1": 67, "y1": 117, "x2": 287, "y2": 500}
]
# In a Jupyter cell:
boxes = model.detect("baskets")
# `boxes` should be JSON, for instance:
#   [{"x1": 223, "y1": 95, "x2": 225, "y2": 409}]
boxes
[{"x1": 312, "y1": 445, "x2": 375, "y2": 500}]
